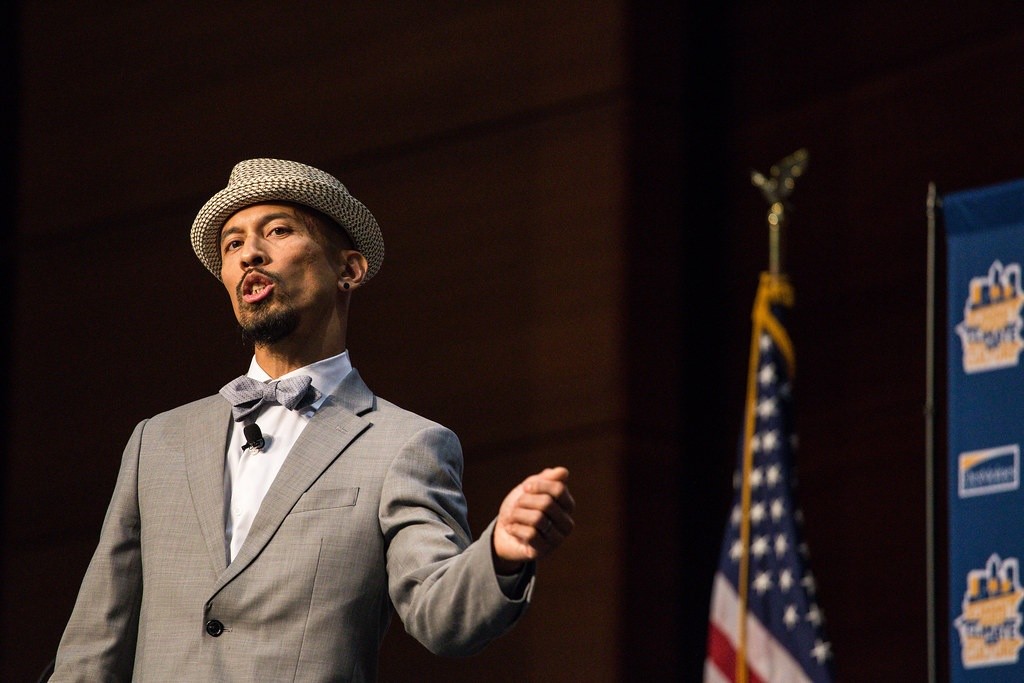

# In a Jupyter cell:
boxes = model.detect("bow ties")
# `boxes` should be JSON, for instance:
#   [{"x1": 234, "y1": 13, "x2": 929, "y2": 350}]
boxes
[{"x1": 219, "y1": 375, "x2": 313, "y2": 422}]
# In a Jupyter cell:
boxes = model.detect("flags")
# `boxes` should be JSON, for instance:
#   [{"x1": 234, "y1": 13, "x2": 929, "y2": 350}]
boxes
[{"x1": 701, "y1": 280, "x2": 830, "y2": 682}]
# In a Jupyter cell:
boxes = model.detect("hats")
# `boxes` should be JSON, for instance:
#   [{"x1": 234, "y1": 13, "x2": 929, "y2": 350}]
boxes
[{"x1": 190, "y1": 159, "x2": 383, "y2": 288}]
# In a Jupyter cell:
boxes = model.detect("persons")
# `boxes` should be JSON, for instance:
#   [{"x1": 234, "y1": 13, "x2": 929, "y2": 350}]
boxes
[{"x1": 45, "y1": 160, "x2": 576, "y2": 683}]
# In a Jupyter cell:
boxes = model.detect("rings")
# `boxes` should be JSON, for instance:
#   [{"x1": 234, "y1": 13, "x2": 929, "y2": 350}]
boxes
[{"x1": 540, "y1": 520, "x2": 552, "y2": 534}]
